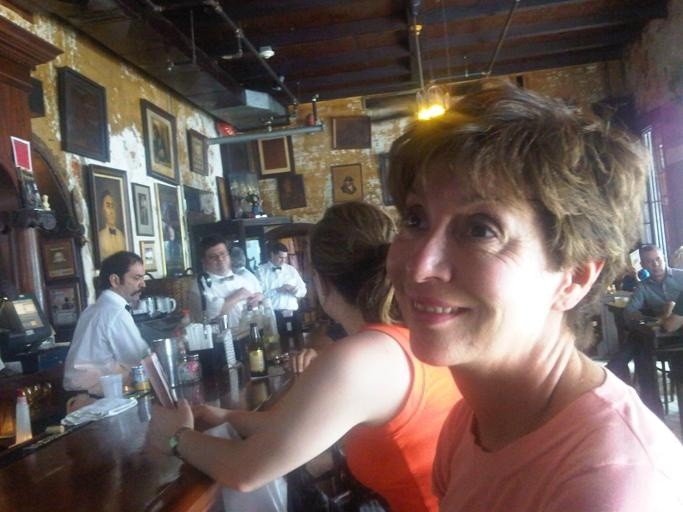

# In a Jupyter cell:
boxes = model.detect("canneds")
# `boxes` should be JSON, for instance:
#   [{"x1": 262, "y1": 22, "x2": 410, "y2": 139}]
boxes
[{"x1": 132, "y1": 365, "x2": 150, "y2": 391}]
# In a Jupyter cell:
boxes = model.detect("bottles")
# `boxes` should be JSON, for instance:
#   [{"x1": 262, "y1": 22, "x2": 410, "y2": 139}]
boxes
[
  {"x1": 175, "y1": 331, "x2": 188, "y2": 358},
  {"x1": 14, "y1": 391, "x2": 34, "y2": 444},
  {"x1": 179, "y1": 353, "x2": 202, "y2": 383},
  {"x1": 247, "y1": 323, "x2": 268, "y2": 377}
]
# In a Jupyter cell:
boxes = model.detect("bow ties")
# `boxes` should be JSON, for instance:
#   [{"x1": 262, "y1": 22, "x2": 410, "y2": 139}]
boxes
[
  {"x1": 272, "y1": 266, "x2": 281, "y2": 271},
  {"x1": 219, "y1": 275, "x2": 234, "y2": 283}
]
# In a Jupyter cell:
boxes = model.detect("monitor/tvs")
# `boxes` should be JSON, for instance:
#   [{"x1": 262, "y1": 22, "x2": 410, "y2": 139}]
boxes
[{"x1": 0, "y1": 293, "x2": 53, "y2": 345}]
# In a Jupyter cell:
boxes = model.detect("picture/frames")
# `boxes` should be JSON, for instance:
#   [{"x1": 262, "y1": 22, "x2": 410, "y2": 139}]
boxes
[
  {"x1": 256, "y1": 114, "x2": 372, "y2": 211},
  {"x1": 38, "y1": 235, "x2": 80, "y2": 334},
  {"x1": 8, "y1": 136, "x2": 41, "y2": 212},
  {"x1": 136, "y1": 94, "x2": 209, "y2": 186},
  {"x1": 56, "y1": 64, "x2": 117, "y2": 165},
  {"x1": 80, "y1": 167, "x2": 185, "y2": 284}
]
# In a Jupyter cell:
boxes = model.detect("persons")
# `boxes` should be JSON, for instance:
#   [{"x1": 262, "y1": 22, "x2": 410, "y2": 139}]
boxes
[
  {"x1": 187, "y1": 233, "x2": 268, "y2": 376},
  {"x1": 135, "y1": 198, "x2": 465, "y2": 511},
  {"x1": 608, "y1": 248, "x2": 649, "y2": 292},
  {"x1": 251, "y1": 243, "x2": 309, "y2": 334},
  {"x1": 290, "y1": 323, "x2": 350, "y2": 375},
  {"x1": 602, "y1": 243, "x2": 683, "y2": 422},
  {"x1": 383, "y1": 71, "x2": 681, "y2": 512},
  {"x1": 662, "y1": 293, "x2": 683, "y2": 420},
  {"x1": 97, "y1": 189, "x2": 126, "y2": 259},
  {"x1": 228, "y1": 244, "x2": 280, "y2": 340},
  {"x1": 49, "y1": 249, "x2": 158, "y2": 425},
  {"x1": 161, "y1": 224, "x2": 183, "y2": 263}
]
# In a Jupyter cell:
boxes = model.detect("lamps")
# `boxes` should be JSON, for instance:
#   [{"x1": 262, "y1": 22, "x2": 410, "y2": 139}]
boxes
[
  {"x1": 411, "y1": 21, "x2": 450, "y2": 120},
  {"x1": 258, "y1": 44, "x2": 274, "y2": 61}
]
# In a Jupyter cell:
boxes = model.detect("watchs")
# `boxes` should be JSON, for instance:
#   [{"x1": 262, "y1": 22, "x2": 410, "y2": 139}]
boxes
[{"x1": 167, "y1": 426, "x2": 194, "y2": 462}]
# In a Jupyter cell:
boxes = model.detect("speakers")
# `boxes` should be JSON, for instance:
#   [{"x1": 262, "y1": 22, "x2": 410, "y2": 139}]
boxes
[{"x1": 591, "y1": 94, "x2": 641, "y2": 144}]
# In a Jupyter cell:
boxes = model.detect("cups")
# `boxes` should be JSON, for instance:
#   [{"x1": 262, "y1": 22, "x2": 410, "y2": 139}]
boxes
[
  {"x1": 136, "y1": 296, "x2": 176, "y2": 315},
  {"x1": 98, "y1": 374, "x2": 123, "y2": 402}
]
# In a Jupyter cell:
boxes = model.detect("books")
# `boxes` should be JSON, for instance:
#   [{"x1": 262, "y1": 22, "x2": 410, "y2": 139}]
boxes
[{"x1": 612, "y1": 249, "x2": 642, "y2": 268}]
